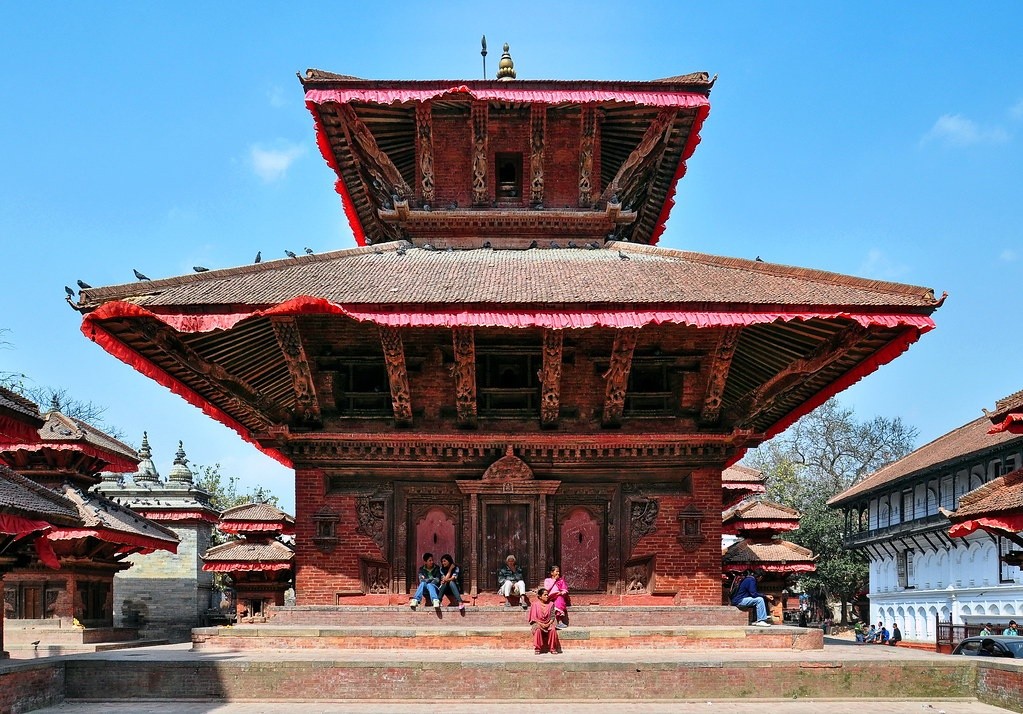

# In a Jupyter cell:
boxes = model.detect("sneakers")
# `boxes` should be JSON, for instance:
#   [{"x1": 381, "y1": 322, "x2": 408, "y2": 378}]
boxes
[
  {"x1": 410, "y1": 599, "x2": 418, "y2": 607},
  {"x1": 433, "y1": 601, "x2": 439, "y2": 607}
]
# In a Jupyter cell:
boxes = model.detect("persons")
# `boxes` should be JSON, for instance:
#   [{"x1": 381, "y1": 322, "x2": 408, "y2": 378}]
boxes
[
  {"x1": 410, "y1": 553, "x2": 464, "y2": 610},
  {"x1": 544, "y1": 566, "x2": 569, "y2": 628},
  {"x1": 854, "y1": 618, "x2": 902, "y2": 646},
  {"x1": 791, "y1": 608, "x2": 834, "y2": 635},
  {"x1": 498, "y1": 555, "x2": 528, "y2": 608},
  {"x1": 729, "y1": 568, "x2": 774, "y2": 627},
  {"x1": 980, "y1": 620, "x2": 1019, "y2": 658},
  {"x1": 529, "y1": 589, "x2": 560, "y2": 654}
]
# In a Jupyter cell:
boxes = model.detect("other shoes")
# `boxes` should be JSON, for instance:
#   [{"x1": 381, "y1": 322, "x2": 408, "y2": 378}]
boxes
[
  {"x1": 522, "y1": 601, "x2": 528, "y2": 607},
  {"x1": 548, "y1": 651, "x2": 558, "y2": 654},
  {"x1": 752, "y1": 622, "x2": 757, "y2": 625},
  {"x1": 756, "y1": 620, "x2": 771, "y2": 627},
  {"x1": 506, "y1": 602, "x2": 511, "y2": 607},
  {"x1": 535, "y1": 651, "x2": 541, "y2": 655},
  {"x1": 459, "y1": 605, "x2": 464, "y2": 609},
  {"x1": 558, "y1": 624, "x2": 567, "y2": 628}
]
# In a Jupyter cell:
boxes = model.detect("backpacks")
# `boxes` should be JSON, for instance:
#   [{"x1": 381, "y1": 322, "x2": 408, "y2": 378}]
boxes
[{"x1": 729, "y1": 576, "x2": 756, "y2": 596}]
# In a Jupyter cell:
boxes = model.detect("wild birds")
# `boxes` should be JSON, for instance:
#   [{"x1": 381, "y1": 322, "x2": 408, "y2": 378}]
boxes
[
  {"x1": 254, "y1": 246, "x2": 315, "y2": 264},
  {"x1": 133, "y1": 268, "x2": 151, "y2": 282},
  {"x1": 193, "y1": 266, "x2": 210, "y2": 273},
  {"x1": 31, "y1": 640, "x2": 42, "y2": 646},
  {"x1": 50, "y1": 422, "x2": 222, "y2": 530},
  {"x1": 756, "y1": 256, "x2": 764, "y2": 263},
  {"x1": 365, "y1": 195, "x2": 635, "y2": 261},
  {"x1": 65, "y1": 279, "x2": 92, "y2": 300}
]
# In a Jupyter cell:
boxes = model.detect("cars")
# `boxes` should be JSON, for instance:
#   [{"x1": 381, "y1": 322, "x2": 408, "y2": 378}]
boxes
[{"x1": 951, "y1": 634, "x2": 1023, "y2": 659}]
